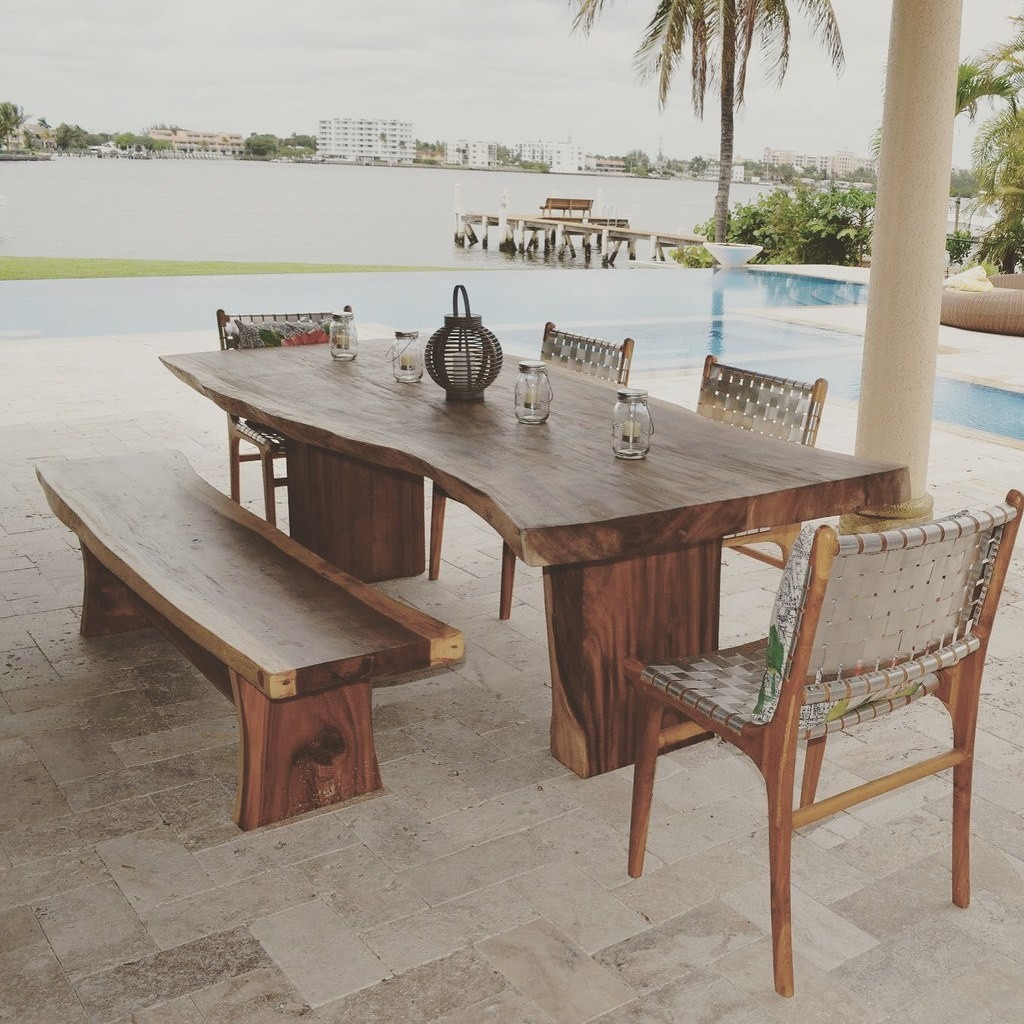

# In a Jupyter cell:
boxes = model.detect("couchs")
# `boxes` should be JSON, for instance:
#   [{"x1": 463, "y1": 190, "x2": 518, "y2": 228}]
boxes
[{"x1": 940, "y1": 265, "x2": 1024, "y2": 336}]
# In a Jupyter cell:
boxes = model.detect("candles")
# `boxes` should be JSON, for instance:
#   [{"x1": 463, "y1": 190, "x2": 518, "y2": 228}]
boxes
[
  {"x1": 622, "y1": 414, "x2": 641, "y2": 439},
  {"x1": 452, "y1": 352, "x2": 475, "y2": 387},
  {"x1": 337, "y1": 329, "x2": 349, "y2": 346},
  {"x1": 402, "y1": 353, "x2": 416, "y2": 366},
  {"x1": 524, "y1": 383, "x2": 542, "y2": 406}
]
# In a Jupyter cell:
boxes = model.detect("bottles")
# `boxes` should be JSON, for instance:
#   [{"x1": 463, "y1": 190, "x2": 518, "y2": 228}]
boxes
[
  {"x1": 328, "y1": 311, "x2": 358, "y2": 361},
  {"x1": 384, "y1": 329, "x2": 424, "y2": 384},
  {"x1": 516, "y1": 361, "x2": 554, "y2": 424},
  {"x1": 613, "y1": 388, "x2": 654, "y2": 460}
]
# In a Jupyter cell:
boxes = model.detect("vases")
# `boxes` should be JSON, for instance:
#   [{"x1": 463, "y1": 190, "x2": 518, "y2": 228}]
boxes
[{"x1": 702, "y1": 241, "x2": 763, "y2": 266}]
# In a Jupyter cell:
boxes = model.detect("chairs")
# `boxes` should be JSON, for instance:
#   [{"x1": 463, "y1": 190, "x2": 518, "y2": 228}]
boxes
[
  {"x1": 429, "y1": 322, "x2": 635, "y2": 619},
  {"x1": 622, "y1": 489, "x2": 1024, "y2": 998},
  {"x1": 722, "y1": 355, "x2": 828, "y2": 570},
  {"x1": 216, "y1": 305, "x2": 352, "y2": 528}
]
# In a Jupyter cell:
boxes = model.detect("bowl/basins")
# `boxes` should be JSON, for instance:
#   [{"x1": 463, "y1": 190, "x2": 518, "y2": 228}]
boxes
[{"x1": 704, "y1": 242, "x2": 764, "y2": 268}]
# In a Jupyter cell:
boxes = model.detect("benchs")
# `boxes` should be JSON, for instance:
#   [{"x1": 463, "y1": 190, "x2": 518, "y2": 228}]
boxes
[
  {"x1": 35, "y1": 450, "x2": 466, "y2": 831},
  {"x1": 539, "y1": 197, "x2": 594, "y2": 217}
]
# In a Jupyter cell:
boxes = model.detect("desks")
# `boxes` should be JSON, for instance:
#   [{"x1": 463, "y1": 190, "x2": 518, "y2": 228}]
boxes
[{"x1": 159, "y1": 333, "x2": 909, "y2": 780}]
